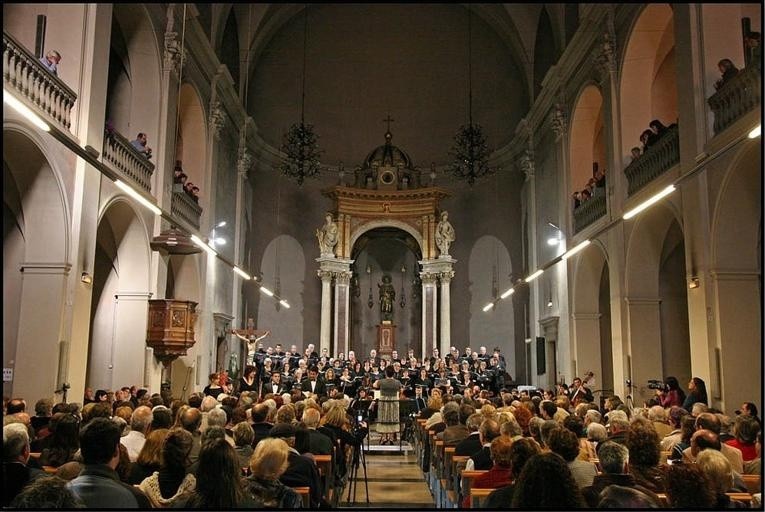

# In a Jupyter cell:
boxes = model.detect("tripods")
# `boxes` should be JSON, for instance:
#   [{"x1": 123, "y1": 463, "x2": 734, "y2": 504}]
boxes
[{"x1": 347, "y1": 424, "x2": 370, "y2": 505}]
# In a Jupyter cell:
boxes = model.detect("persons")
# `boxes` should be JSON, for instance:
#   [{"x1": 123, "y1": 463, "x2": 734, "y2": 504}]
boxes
[
  {"x1": 712, "y1": 59, "x2": 740, "y2": 92},
  {"x1": 174, "y1": 164, "x2": 199, "y2": 204},
  {"x1": 373, "y1": 365, "x2": 404, "y2": 446},
  {"x1": 377, "y1": 273, "x2": 397, "y2": 321},
  {"x1": 1, "y1": 369, "x2": 376, "y2": 511},
  {"x1": 240, "y1": 344, "x2": 507, "y2": 410},
  {"x1": 434, "y1": 210, "x2": 456, "y2": 254},
  {"x1": 130, "y1": 133, "x2": 152, "y2": 160},
  {"x1": 572, "y1": 167, "x2": 604, "y2": 210},
  {"x1": 404, "y1": 375, "x2": 761, "y2": 511},
  {"x1": 234, "y1": 327, "x2": 270, "y2": 367},
  {"x1": 629, "y1": 118, "x2": 678, "y2": 165},
  {"x1": 316, "y1": 211, "x2": 340, "y2": 258},
  {"x1": 38, "y1": 49, "x2": 61, "y2": 77},
  {"x1": 744, "y1": 30, "x2": 762, "y2": 67}
]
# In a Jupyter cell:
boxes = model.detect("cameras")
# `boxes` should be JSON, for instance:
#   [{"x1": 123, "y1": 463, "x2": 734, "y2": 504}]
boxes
[{"x1": 356, "y1": 415, "x2": 363, "y2": 424}]
